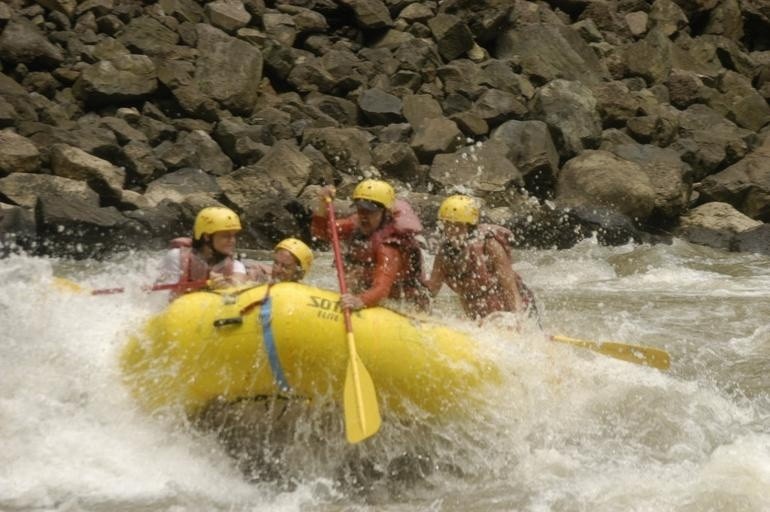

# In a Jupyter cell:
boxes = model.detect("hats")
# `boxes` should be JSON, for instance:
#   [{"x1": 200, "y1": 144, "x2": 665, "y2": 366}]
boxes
[{"x1": 349, "y1": 198, "x2": 383, "y2": 212}]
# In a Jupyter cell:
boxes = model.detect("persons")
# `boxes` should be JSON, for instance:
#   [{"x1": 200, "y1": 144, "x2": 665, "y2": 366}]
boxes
[
  {"x1": 151, "y1": 205, "x2": 246, "y2": 317},
  {"x1": 412, "y1": 192, "x2": 545, "y2": 336},
  {"x1": 308, "y1": 176, "x2": 431, "y2": 317},
  {"x1": 244, "y1": 236, "x2": 314, "y2": 286}
]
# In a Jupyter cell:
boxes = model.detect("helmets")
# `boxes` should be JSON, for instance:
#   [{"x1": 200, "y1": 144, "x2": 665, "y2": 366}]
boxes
[
  {"x1": 275, "y1": 238, "x2": 315, "y2": 281},
  {"x1": 352, "y1": 177, "x2": 395, "y2": 212},
  {"x1": 194, "y1": 205, "x2": 242, "y2": 240},
  {"x1": 436, "y1": 194, "x2": 480, "y2": 225}
]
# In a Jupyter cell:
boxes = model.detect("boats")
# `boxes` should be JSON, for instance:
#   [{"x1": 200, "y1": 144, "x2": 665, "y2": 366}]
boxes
[{"x1": 110, "y1": 282, "x2": 568, "y2": 491}]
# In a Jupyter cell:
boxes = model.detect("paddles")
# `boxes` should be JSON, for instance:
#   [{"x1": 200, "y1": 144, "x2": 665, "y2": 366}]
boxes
[
  {"x1": 418, "y1": 316, "x2": 671, "y2": 374},
  {"x1": 39, "y1": 275, "x2": 213, "y2": 301},
  {"x1": 325, "y1": 197, "x2": 383, "y2": 445}
]
